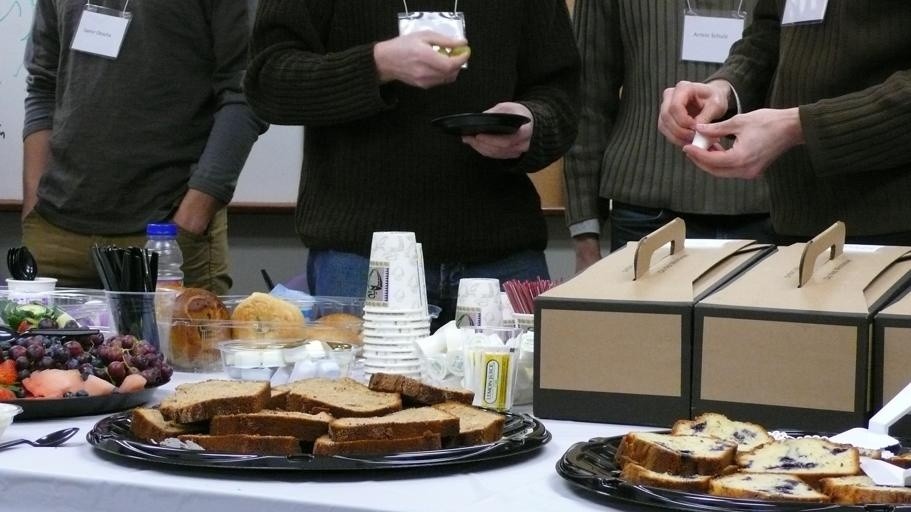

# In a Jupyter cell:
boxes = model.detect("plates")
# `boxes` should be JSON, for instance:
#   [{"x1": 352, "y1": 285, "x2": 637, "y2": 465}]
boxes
[
  {"x1": 86, "y1": 403, "x2": 554, "y2": 470},
  {"x1": 0, "y1": 379, "x2": 171, "y2": 420},
  {"x1": 556, "y1": 426, "x2": 911, "y2": 512},
  {"x1": 430, "y1": 112, "x2": 532, "y2": 136}
]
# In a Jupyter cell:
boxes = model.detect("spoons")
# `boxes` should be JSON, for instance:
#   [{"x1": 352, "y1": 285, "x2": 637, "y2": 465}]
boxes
[
  {"x1": 0, "y1": 427, "x2": 79, "y2": 449},
  {"x1": 6, "y1": 246, "x2": 38, "y2": 281}
]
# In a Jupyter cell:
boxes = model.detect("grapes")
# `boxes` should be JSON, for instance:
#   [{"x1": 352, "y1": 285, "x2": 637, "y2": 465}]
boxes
[{"x1": 0, "y1": 318, "x2": 173, "y2": 397}]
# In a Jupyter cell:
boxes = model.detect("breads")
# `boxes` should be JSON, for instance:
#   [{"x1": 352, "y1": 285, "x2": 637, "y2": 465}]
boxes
[
  {"x1": 309, "y1": 313, "x2": 363, "y2": 342},
  {"x1": 614, "y1": 412, "x2": 911, "y2": 505},
  {"x1": 170, "y1": 288, "x2": 231, "y2": 368},
  {"x1": 231, "y1": 292, "x2": 307, "y2": 341},
  {"x1": 130, "y1": 372, "x2": 504, "y2": 456}
]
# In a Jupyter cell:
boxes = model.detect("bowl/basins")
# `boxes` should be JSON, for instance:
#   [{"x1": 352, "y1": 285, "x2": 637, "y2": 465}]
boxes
[
  {"x1": 0, "y1": 401, "x2": 24, "y2": 439},
  {"x1": 154, "y1": 295, "x2": 443, "y2": 386}
]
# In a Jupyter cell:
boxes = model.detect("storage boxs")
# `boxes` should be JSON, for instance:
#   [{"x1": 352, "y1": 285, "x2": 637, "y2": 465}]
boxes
[{"x1": 533, "y1": 216, "x2": 911, "y2": 431}]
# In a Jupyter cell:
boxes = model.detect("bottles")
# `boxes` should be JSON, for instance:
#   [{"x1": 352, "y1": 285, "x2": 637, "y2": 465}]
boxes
[{"x1": 142, "y1": 222, "x2": 184, "y2": 292}]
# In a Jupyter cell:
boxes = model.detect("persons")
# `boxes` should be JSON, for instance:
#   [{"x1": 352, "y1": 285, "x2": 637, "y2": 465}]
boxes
[
  {"x1": 242, "y1": 0, "x2": 584, "y2": 322},
  {"x1": 656, "y1": 0, "x2": 911, "y2": 243},
  {"x1": 560, "y1": 0, "x2": 774, "y2": 278},
  {"x1": 16, "y1": 0, "x2": 272, "y2": 297}
]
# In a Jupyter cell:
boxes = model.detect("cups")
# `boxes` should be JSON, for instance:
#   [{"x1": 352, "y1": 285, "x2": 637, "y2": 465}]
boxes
[
  {"x1": 453, "y1": 276, "x2": 538, "y2": 416},
  {"x1": 3, "y1": 275, "x2": 58, "y2": 308},
  {"x1": 104, "y1": 287, "x2": 177, "y2": 370},
  {"x1": 359, "y1": 229, "x2": 432, "y2": 384}
]
